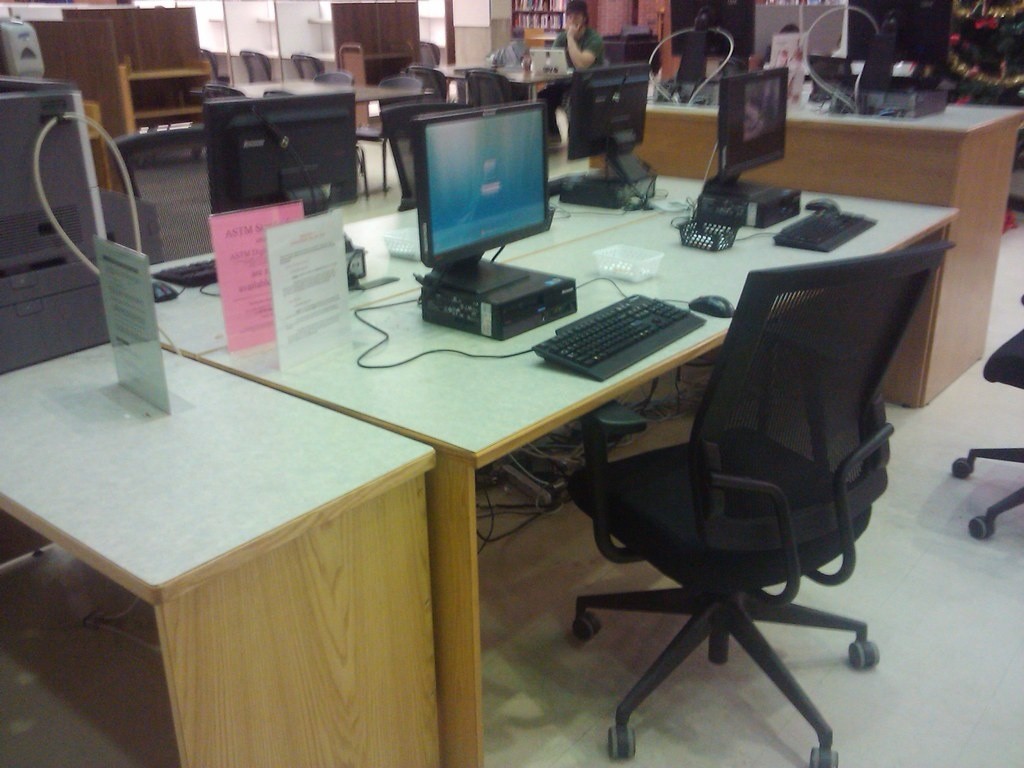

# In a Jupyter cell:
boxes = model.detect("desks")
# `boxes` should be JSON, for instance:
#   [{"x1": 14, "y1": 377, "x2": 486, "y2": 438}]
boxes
[
  {"x1": 0, "y1": 341, "x2": 438, "y2": 767},
  {"x1": 226, "y1": 81, "x2": 426, "y2": 115},
  {"x1": 141, "y1": 176, "x2": 957, "y2": 768},
  {"x1": 435, "y1": 64, "x2": 575, "y2": 103}
]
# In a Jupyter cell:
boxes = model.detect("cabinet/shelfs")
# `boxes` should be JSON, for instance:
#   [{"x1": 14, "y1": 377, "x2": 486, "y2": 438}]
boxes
[
  {"x1": 113, "y1": 60, "x2": 214, "y2": 137},
  {"x1": 510, "y1": 0, "x2": 580, "y2": 32},
  {"x1": 339, "y1": 44, "x2": 415, "y2": 137}
]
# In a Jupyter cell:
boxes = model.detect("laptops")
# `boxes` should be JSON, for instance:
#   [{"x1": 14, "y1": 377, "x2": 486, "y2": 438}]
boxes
[{"x1": 528, "y1": 46, "x2": 575, "y2": 75}]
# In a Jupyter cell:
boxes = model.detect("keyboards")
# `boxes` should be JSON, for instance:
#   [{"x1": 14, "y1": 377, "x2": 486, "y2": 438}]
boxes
[
  {"x1": 153, "y1": 260, "x2": 222, "y2": 288},
  {"x1": 698, "y1": 184, "x2": 802, "y2": 229},
  {"x1": 776, "y1": 211, "x2": 876, "y2": 252},
  {"x1": 532, "y1": 292, "x2": 707, "y2": 382},
  {"x1": 546, "y1": 178, "x2": 570, "y2": 197}
]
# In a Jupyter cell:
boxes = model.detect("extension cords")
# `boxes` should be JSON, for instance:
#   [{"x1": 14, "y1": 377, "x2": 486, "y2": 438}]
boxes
[{"x1": 501, "y1": 464, "x2": 553, "y2": 507}]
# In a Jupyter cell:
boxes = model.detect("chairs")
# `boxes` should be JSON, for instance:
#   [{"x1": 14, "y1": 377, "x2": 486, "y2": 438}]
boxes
[
  {"x1": 380, "y1": 104, "x2": 445, "y2": 211},
  {"x1": 953, "y1": 329, "x2": 1024, "y2": 541},
  {"x1": 110, "y1": 127, "x2": 217, "y2": 265},
  {"x1": 191, "y1": 49, "x2": 609, "y2": 102},
  {"x1": 570, "y1": 243, "x2": 956, "y2": 768}
]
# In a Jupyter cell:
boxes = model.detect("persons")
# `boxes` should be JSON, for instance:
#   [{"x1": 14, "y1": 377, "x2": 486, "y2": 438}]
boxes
[{"x1": 551, "y1": 0, "x2": 606, "y2": 144}]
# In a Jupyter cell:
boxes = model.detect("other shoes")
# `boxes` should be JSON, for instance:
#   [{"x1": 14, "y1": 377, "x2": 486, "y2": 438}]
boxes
[{"x1": 546, "y1": 135, "x2": 561, "y2": 150}]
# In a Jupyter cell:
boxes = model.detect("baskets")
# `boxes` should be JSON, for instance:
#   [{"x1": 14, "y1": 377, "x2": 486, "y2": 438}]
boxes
[{"x1": 677, "y1": 218, "x2": 737, "y2": 251}]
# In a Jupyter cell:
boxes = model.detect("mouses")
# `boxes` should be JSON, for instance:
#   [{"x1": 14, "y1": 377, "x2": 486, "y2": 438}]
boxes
[
  {"x1": 689, "y1": 296, "x2": 738, "y2": 318},
  {"x1": 806, "y1": 197, "x2": 842, "y2": 213},
  {"x1": 154, "y1": 283, "x2": 177, "y2": 303}
]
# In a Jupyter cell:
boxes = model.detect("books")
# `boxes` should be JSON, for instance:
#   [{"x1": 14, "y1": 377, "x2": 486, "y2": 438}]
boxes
[{"x1": 513, "y1": 0, "x2": 565, "y2": 29}]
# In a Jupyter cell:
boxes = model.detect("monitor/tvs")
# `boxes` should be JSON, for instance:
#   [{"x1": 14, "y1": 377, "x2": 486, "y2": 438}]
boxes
[
  {"x1": 709, "y1": 67, "x2": 792, "y2": 196},
  {"x1": 204, "y1": 94, "x2": 358, "y2": 221},
  {"x1": 568, "y1": 63, "x2": 650, "y2": 184},
  {"x1": 408, "y1": 100, "x2": 551, "y2": 296}
]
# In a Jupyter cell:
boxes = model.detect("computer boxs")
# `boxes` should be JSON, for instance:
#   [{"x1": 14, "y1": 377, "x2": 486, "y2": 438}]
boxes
[
  {"x1": 421, "y1": 270, "x2": 578, "y2": 341},
  {"x1": 558, "y1": 172, "x2": 657, "y2": 209}
]
